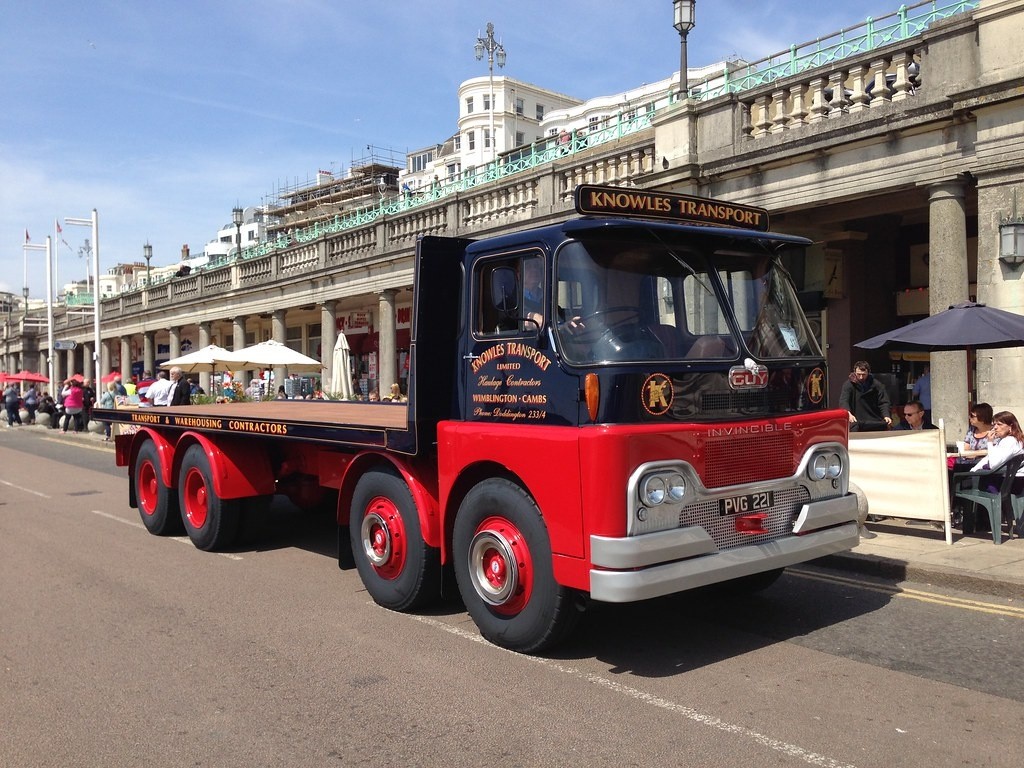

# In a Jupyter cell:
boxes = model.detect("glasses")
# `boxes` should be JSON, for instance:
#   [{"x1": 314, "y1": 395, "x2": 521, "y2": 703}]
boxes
[
  {"x1": 903, "y1": 411, "x2": 920, "y2": 417},
  {"x1": 969, "y1": 413, "x2": 978, "y2": 419}
]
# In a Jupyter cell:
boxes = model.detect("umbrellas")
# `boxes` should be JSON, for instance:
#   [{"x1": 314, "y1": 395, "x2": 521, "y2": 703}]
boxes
[
  {"x1": 854, "y1": 300, "x2": 1024, "y2": 429},
  {"x1": 94, "y1": 371, "x2": 136, "y2": 383},
  {"x1": 331, "y1": 333, "x2": 354, "y2": 401},
  {"x1": 0, "y1": 370, "x2": 49, "y2": 383},
  {"x1": 64, "y1": 372, "x2": 83, "y2": 384},
  {"x1": 160, "y1": 345, "x2": 255, "y2": 395},
  {"x1": 214, "y1": 338, "x2": 327, "y2": 396}
]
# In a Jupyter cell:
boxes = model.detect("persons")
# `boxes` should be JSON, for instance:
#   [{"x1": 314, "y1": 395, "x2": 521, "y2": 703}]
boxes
[
  {"x1": 912, "y1": 366, "x2": 934, "y2": 429},
  {"x1": 839, "y1": 360, "x2": 892, "y2": 425},
  {"x1": 246, "y1": 378, "x2": 324, "y2": 400},
  {"x1": 496, "y1": 258, "x2": 585, "y2": 334},
  {"x1": 891, "y1": 401, "x2": 938, "y2": 430},
  {"x1": 352, "y1": 374, "x2": 407, "y2": 402},
  {"x1": 2, "y1": 382, "x2": 65, "y2": 429},
  {"x1": 951, "y1": 411, "x2": 1024, "y2": 527},
  {"x1": 135, "y1": 368, "x2": 205, "y2": 408},
  {"x1": 57, "y1": 377, "x2": 136, "y2": 441},
  {"x1": 964, "y1": 402, "x2": 1001, "y2": 465}
]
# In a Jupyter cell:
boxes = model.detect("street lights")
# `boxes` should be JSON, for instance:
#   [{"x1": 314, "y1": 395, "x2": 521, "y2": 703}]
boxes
[
  {"x1": 673, "y1": 0, "x2": 696, "y2": 102},
  {"x1": 233, "y1": 199, "x2": 244, "y2": 263},
  {"x1": 23, "y1": 287, "x2": 29, "y2": 315},
  {"x1": 474, "y1": 21, "x2": 508, "y2": 172},
  {"x1": 6, "y1": 293, "x2": 13, "y2": 324},
  {"x1": 77, "y1": 237, "x2": 92, "y2": 294},
  {"x1": 143, "y1": 239, "x2": 153, "y2": 287}
]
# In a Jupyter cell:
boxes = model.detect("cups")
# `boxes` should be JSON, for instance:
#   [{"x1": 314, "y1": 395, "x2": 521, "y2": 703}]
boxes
[{"x1": 956, "y1": 442, "x2": 964, "y2": 452}]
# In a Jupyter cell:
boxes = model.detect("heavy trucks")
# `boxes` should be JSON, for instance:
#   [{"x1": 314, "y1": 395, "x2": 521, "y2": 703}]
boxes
[{"x1": 89, "y1": 184, "x2": 859, "y2": 657}]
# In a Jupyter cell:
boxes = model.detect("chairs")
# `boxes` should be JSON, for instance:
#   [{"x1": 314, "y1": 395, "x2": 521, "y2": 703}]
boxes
[
  {"x1": 646, "y1": 324, "x2": 680, "y2": 355},
  {"x1": 943, "y1": 452, "x2": 1024, "y2": 545}
]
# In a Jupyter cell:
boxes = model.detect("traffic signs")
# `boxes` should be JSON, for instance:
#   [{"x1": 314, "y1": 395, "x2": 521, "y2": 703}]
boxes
[{"x1": 55, "y1": 340, "x2": 78, "y2": 350}]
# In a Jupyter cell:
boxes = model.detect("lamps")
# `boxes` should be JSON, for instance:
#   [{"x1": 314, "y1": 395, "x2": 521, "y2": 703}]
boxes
[{"x1": 998, "y1": 214, "x2": 1024, "y2": 270}]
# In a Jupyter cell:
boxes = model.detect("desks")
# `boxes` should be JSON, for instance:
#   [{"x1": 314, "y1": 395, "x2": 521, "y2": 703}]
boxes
[{"x1": 947, "y1": 450, "x2": 988, "y2": 530}]
